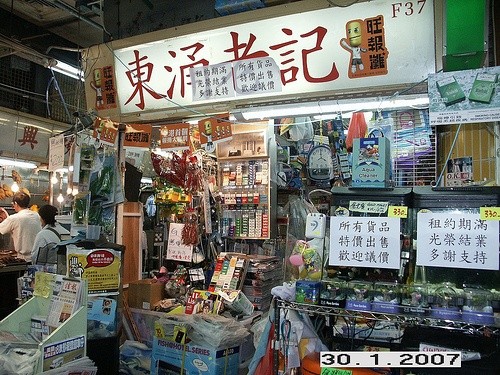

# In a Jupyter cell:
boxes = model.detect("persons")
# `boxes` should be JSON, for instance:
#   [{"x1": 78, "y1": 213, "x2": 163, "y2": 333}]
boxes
[
  {"x1": 0, "y1": 191, "x2": 42, "y2": 264},
  {"x1": 29, "y1": 204, "x2": 72, "y2": 264}
]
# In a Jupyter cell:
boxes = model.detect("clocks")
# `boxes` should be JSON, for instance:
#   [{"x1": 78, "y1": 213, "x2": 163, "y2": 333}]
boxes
[{"x1": 307, "y1": 144, "x2": 333, "y2": 182}]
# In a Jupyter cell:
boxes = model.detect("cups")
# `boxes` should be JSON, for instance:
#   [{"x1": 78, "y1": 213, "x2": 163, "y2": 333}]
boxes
[{"x1": 86, "y1": 225, "x2": 101, "y2": 240}]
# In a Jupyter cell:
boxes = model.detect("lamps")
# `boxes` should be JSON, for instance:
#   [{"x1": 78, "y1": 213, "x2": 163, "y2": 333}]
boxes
[
  {"x1": 47, "y1": 58, "x2": 85, "y2": 81},
  {"x1": 230, "y1": 93, "x2": 431, "y2": 121}
]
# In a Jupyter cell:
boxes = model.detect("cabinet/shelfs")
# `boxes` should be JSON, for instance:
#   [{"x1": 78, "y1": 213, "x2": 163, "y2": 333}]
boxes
[
  {"x1": 216, "y1": 118, "x2": 279, "y2": 240},
  {"x1": 276, "y1": 184, "x2": 297, "y2": 226}
]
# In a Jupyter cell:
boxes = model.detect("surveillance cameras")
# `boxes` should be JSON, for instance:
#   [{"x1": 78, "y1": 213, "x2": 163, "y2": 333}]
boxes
[{"x1": 72, "y1": 111, "x2": 88, "y2": 119}]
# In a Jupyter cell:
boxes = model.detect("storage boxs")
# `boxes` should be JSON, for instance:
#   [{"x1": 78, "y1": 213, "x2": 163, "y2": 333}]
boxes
[
  {"x1": 87, "y1": 252, "x2": 264, "y2": 375},
  {"x1": 214, "y1": 0, "x2": 265, "y2": 16},
  {"x1": 295, "y1": 279, "x2": 320, "y2": 305},
  {"x1": 351, "y1": 136, "x2": 392, "y2": 189}
]
